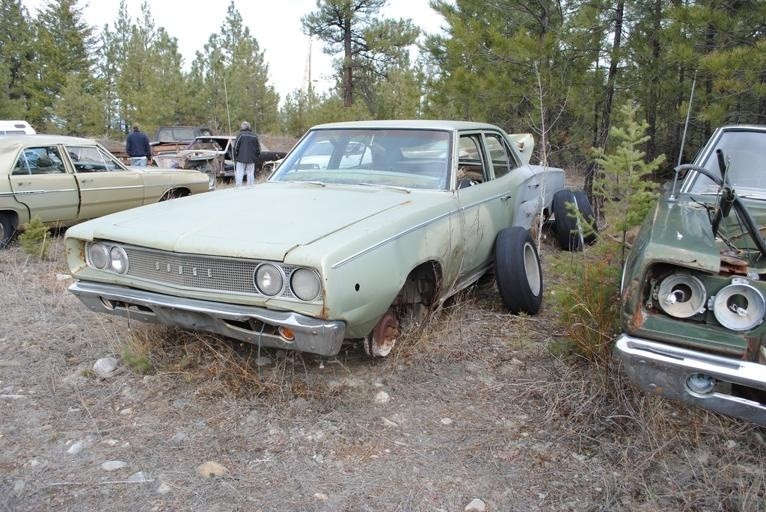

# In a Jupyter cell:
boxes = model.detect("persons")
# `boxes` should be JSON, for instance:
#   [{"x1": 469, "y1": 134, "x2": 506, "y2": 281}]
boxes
[
  {"x1": 125, "y1": 123, "x2": 153, "y2": 168},
  {"x1": 232, "y1": 121, "x2": 260, "y2": 186}
]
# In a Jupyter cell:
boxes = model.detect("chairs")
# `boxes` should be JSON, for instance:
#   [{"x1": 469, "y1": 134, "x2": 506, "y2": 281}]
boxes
[
  {"x1": 371, "y1": 141, "x2": 406, "y2": 172},
  {"x1": 13, "y1": 156, "x2": 57, "y2": 174}
]
